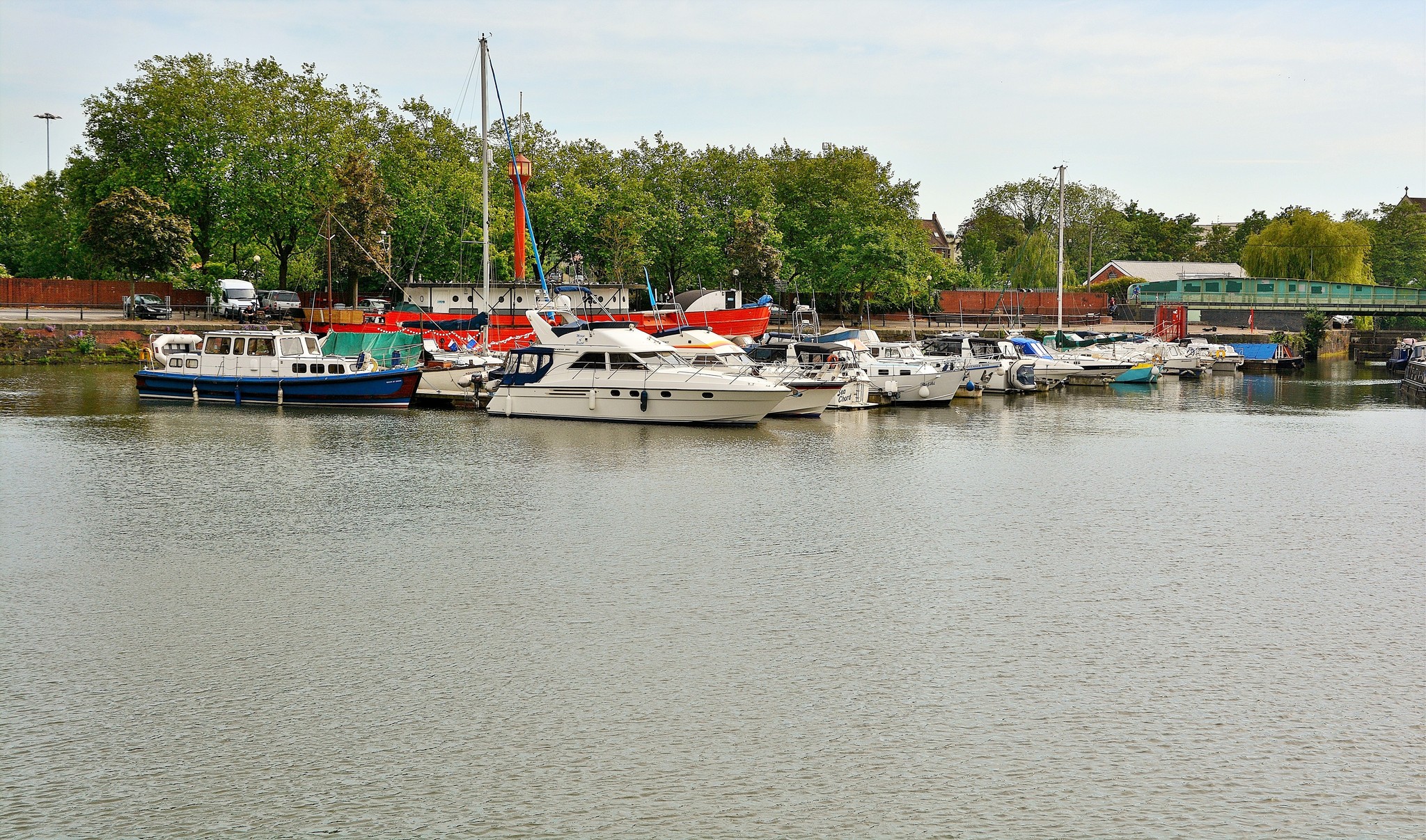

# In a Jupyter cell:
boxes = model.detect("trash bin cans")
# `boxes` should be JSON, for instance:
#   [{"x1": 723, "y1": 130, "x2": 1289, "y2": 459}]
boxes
[{"x1": 334, "y1": 304, "x2": 345, "y2": 309}]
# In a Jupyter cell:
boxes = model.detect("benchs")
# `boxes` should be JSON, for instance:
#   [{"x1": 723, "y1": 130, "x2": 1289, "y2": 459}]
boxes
[
  {"x1": 936, "y1": 318, "x2": 963, "y2": 327},
  {"x1": 241, "y1": 308, "x2": 267, "y2": 324}
]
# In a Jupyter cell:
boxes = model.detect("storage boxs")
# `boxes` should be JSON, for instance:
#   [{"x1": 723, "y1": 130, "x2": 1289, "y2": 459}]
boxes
[{"x1": 428, "y1": 360, "x2": 451, "y2": 368}]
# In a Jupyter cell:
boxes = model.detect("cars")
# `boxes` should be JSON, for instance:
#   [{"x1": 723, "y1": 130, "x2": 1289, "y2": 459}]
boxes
[
  {"x1": 350, "y1": 298, "x2": 395, "y2": 323},
  {"x1": 766, "y1": 304, "x2": 789, "y2": 325},
  {"x1": 124, "y1": 294, "x2": 172, "y2": 320}
]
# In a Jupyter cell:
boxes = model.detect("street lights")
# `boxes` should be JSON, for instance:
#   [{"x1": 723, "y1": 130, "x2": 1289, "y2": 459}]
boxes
[
  {"x1": 253, "y1": 255, "x2": 261, "y2": 324},
  {"x1": 33, "y1": 112, "x2": 63, "y2": 173}
]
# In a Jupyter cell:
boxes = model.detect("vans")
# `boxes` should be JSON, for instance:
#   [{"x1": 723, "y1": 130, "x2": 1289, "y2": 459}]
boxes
[{"x1": 210, "y1": 278, "x2": 260, "y2": 320}]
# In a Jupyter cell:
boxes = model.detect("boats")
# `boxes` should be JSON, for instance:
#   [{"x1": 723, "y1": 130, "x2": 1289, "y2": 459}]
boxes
[
  {"x1": 1386, "y1": 337, "x2": 1419, "y2": 372},
  {"x1": 1400, "y1": 340, "x2": 1426, "y2": 401},
  {"x1": 359, "y1": 29, "x2": 1308, "y2": 429},
  {"x1": 130, "y1": 206, "x2": 457, "y2": 410},
  {"x1": 486, "y1": 284, "x2": 815, "y2": 427}
]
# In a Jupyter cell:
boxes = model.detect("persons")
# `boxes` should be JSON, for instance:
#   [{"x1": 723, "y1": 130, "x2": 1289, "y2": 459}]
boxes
[
  {"x1": 1108, "y1": 285, "x2": 1141, "y2": 309},
  {"x1": 243, "y1": 305, "x2": 254, "y2": 323}
]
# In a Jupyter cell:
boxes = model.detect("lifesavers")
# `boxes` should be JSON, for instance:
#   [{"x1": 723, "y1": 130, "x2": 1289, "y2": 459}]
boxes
[
  {"x1": 827, "y1": 355, "x2": 838, "y2": 367},
  {"x1": 139, "y1": 347, "x2": 152, "y2": 361},
  {"x1": 546, "y1": 320, "x2": 557, "y2": 326},
  {"x1": 1152, "y1": 354, "x2": 1162, "y2": 363},
  {"x1": 1216, "y1": 349, "x2": 1225, "y2": 358},
  {"x1": 370, "y1": 357, "x2": 378, "y2": 373}
]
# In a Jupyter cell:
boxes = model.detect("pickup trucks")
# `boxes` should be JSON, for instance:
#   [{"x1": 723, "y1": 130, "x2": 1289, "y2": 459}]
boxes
[{"x1": 261, "y1": 290, "x2": 302, "y2": 318}]
